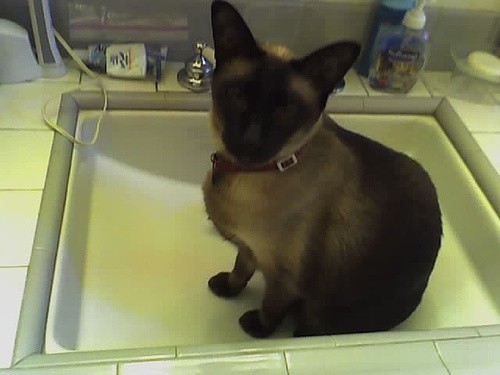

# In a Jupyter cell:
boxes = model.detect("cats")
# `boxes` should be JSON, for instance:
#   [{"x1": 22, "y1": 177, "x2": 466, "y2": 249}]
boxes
[{"x1": 201, "y1": 0, "x2": 442, "y2": 338}]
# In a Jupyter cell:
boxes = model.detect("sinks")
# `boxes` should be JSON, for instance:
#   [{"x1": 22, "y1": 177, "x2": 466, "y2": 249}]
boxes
[{"x1": 54, "y1": 115, "x2": 498, "y2": 350}]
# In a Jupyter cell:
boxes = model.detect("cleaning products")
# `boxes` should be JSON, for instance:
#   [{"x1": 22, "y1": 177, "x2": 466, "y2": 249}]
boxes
[{"x1": 356, "y1": 1, "x2": 416, "y2": 78}]
